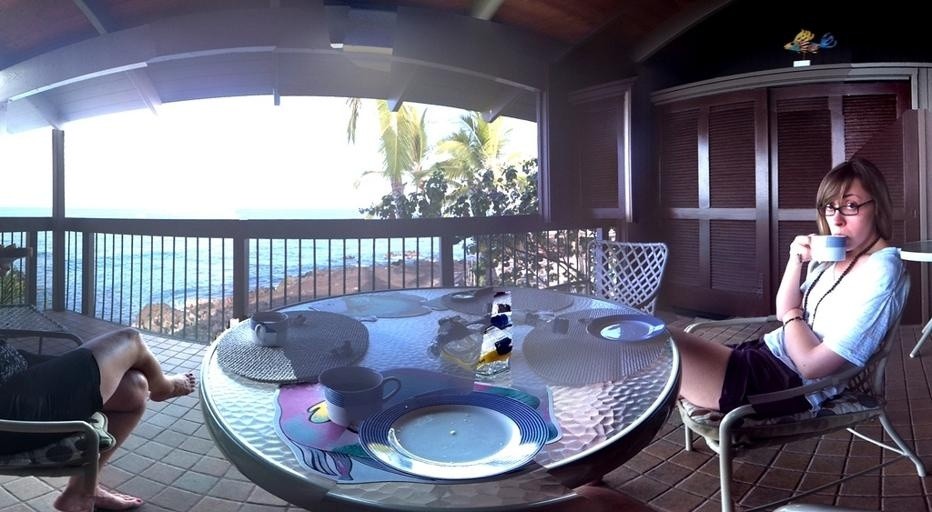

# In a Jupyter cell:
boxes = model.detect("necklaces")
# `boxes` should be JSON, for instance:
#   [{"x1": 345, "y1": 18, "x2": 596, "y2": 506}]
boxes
[{"x1": 803, "y1": 232, "x2": 885, "y2": 329}]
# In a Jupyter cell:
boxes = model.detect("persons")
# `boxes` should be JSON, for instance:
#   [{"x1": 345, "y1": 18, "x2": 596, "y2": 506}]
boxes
[
  {"x1": 0, "y1": 325, "x2": 198, "y2": 511},
  {"x1": 658, "y1": 155, "x2": 908, "y2": 416}
]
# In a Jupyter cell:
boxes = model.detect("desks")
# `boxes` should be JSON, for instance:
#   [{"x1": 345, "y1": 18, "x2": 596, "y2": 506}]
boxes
[
  {"x1": 199, "y1": 287, "x2": 682, "y2": 512},
  {"x1": 898, "y1": 240, "x2": 932, "y2": 325}
]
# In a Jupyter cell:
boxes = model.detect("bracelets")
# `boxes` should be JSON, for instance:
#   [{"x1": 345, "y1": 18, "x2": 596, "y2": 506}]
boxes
[{"x1": 782, "y1": 316, "x2": 807, "y2": 331}]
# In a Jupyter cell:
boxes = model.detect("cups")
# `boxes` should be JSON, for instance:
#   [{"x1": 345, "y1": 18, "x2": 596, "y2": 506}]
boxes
[
  {"x1": 318, "y1": 364, "x2": 402, "y2": 429},
  {"x1": 250, "y1": 312, "x2": 289, "y2": 348},
  {"x1": 804, "y1": 233, "x2": 849, "y2": 263}
]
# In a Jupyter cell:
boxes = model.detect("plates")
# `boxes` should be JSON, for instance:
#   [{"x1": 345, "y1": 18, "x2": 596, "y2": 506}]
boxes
[
  {"x1": 357, "y1": 389, "x2": 550, "y2": 480},
  {"x1": 585, "y1": 313, "x2": 666, "y2": 342}
]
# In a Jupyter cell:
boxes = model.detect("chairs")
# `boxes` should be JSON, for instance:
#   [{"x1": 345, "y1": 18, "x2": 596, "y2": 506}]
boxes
[
  {"x1": 0, "y1": 329, "x2": 116, "y2": 512},
  {"x1": 678, "y1": 293, "x2": 925, "y2": 512},
  {"x1": 546, "y1": 240, "x2": 670, "y2": 316}
]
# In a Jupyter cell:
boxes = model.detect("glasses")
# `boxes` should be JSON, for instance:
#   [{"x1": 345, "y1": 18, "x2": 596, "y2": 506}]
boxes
[{"x1": 817, "y1": 199, "x2": 873, "y2": 215}]
